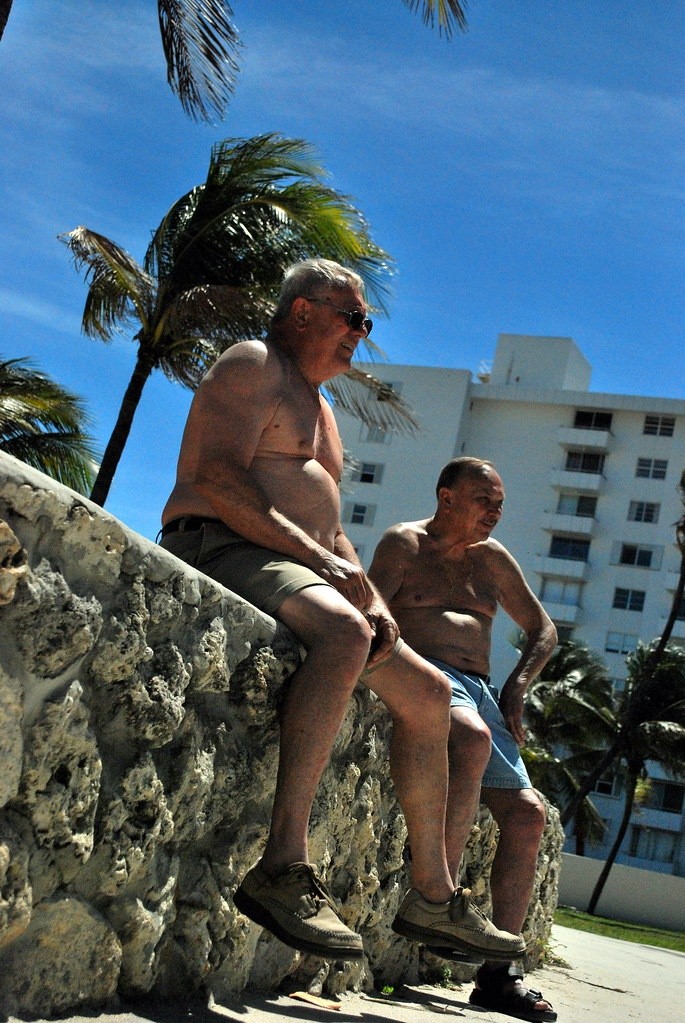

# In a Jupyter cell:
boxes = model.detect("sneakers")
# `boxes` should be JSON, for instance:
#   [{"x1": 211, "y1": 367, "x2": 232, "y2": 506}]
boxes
[
  {"x1": 391, "y1": 886, "x2": 527, "y2": 961},
  {"x1": 233, "y1": 862, "x2": 364, "y2": 961}
]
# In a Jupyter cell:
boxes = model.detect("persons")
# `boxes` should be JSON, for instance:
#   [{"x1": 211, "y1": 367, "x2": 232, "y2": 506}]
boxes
[
  {"x1": 155, "y1": 259, "x2": 527, "y2": 961},
  {"x1": 366, "y1": 457, "x2": 559, "y2": 1023}
]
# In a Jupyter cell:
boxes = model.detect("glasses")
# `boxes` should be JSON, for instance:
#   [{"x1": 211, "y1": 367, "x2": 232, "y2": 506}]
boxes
[{"x1": 306, "y1": 298, "x2": 372, "y2": 339}]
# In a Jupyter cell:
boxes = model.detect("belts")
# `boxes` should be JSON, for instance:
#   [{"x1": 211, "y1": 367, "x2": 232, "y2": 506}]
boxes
[{"x1": 162, "y1": 517, "x2": 222, "y2": 539}]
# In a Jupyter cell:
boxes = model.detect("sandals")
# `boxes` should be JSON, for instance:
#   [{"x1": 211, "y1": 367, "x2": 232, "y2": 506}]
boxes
[
  {"x1": 426, "y1": 944, "x2": 483, "y2": 964},
  {"x1": 469, "y1": 963, "x2": 557, "y2": 1022}
]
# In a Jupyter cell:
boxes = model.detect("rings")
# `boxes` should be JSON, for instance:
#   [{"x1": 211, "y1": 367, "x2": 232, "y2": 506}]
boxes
[{"x1": 514, "y1": 720, "x2": 521, "y2": 724}]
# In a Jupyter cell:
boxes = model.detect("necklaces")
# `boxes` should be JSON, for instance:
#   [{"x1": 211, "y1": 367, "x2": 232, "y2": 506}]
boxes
[
  {"x1": 277, "y1": 341, "x2": 320, "y2": 393},
  {"x1": 432, "y1": 515, "x2": 471, "y2": 594}
]
